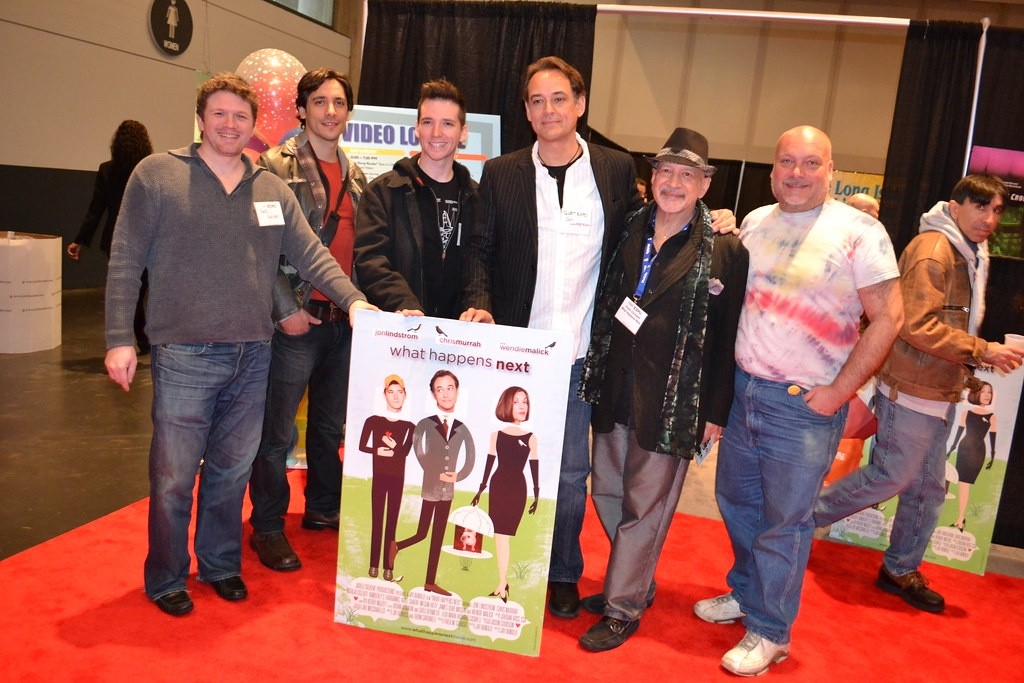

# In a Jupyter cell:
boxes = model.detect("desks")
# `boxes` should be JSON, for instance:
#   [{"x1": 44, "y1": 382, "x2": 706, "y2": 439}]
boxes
[{"x1": 0, "y1": 232, "x2": 62, "y2": 354}]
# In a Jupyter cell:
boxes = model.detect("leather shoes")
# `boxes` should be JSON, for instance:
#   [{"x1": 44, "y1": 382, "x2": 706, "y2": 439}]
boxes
[
  {"x1": 156, "y1": 591, "x2": 193, "y2": 615},
  {"x1": 213, "y1": 575, "x2": 247, "y2": 600},
  {"x1": 548, "y1": 580, "x2": 580, "y2": 618},
  {"x1": 582, "y1": 589, "x2": 654, "y2": 613},
  {"x1": 250, "y1": 525, "x2": 299, "y2": 572},
  {"x1": 303, "y1": 507, "x2": 340, "y2": 530},
  {"x1": 580, "y1": 606, "x2": 638, "y2": 649}
]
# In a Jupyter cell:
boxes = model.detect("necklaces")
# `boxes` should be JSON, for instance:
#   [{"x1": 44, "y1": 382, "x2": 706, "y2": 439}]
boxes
[{"x1": 659, "y1": 229, "x2": 671, "y2": 239}]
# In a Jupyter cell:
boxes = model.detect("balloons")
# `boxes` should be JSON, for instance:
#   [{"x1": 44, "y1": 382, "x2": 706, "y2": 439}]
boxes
[{"x1": 235, "y1": 48, "x2": 310, "y2": 149}]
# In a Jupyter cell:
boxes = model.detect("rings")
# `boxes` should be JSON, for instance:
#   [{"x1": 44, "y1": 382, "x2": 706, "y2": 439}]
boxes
[{"x1": 718, "y1": 435, "x2": 723, "y2": 439}]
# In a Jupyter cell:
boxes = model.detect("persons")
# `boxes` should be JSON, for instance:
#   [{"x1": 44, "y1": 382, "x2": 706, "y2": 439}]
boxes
[
  {"x1": 968, "y1": 381, "x2": 992, "y2": 407},
  {"x1": 459, "y1": 56, "x2": 740, "y2": 619},
  {"x1": 103, "y1": 77, "x2": 378, "y2": 618},
  {"x1": 812, "y1": 172, "x2": 1024, "y2": 612},
  {"x1": 844, "y1": 192, "x2": 880, "y2": 220},
  {"x1": 248, "y1": 67, "x2": 370, "y2": 574},
  {"x1": 576, "y1": 126, "x2": 749, "y2": 655},
  {"x1": 695, "y1": 442, "x2": 709, "y2": 458},
  {"x1": 350, "y1": 77, "x2": 481, "y2": 316},
  {"x1": 430, "y1": 369, "x2": 460, "y2": 413},
  {"x1": 687, "y1": 126, "x2": 904, "y2": 676},
  {"x1": 65, "y1": 120, "x2": 150, "y2": 356},
  {"x1": 383, "y1": 373, "x2": 407, "y2": 414},
  {"x1": 495, "y1": 385, "x2": 530, "y2": 422}
]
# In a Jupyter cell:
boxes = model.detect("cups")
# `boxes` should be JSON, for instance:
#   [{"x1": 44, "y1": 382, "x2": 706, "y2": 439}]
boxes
[{"x1": 1003, "y1": 333, "x2": 1024, "y2": 369}]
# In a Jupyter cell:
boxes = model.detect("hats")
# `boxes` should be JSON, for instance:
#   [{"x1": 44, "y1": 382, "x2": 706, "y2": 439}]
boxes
[{"x1": 641, "y1": 127, "x2": 716, "y2": 178}]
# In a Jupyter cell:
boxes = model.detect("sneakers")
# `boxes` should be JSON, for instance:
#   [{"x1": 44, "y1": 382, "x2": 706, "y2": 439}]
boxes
[
  {"x1": 877, "y1": 563, "x2": 946, "y2": 613},
  {"x1": 695, "y1": 593, "x2": 746, "y2": 624},
  {"x1": 722, "y1": 627, "x2": 788, "y2": 677}
]
